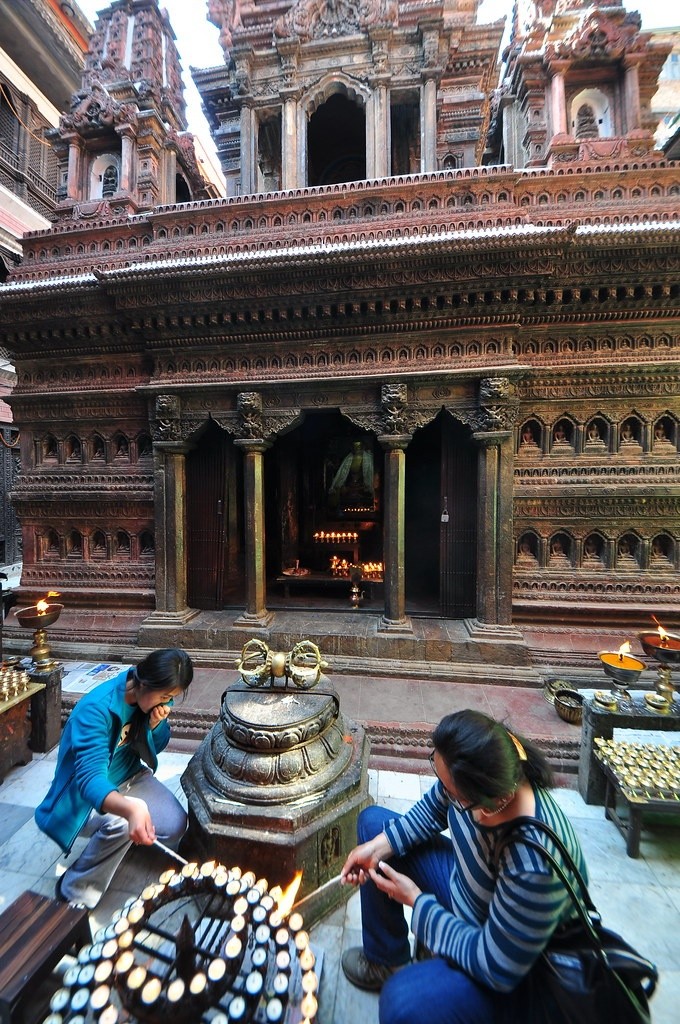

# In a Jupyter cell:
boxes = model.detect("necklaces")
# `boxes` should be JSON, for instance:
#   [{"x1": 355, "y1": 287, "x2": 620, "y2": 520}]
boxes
[{"x1": 481, "y1": 783, "x2": 517, "y2": 817}]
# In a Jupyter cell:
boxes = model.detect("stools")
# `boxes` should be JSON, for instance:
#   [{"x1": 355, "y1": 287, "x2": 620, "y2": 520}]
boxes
[{"x1": 0, "y1": 889, "x2": 93, "y2": 1024}]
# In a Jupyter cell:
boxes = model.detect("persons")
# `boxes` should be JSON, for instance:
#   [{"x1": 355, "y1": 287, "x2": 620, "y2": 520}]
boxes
[
  {"x1": 554, "y1": 423, "x2": 671, "y2": 442},
  {"x1": 328, "y1": 440, "x2": 380, "y2": 487},
  {"x1": 35, "y1": 648, "x2": 194, "y2": 910},
  {"x1": 520, "y1": 537, "x2": 669, "y2": 558},
  {"x1": 522, "y1": 427, "x2": 535, "y2": 445},
  {"x1": 339, "y1": 708, "x2": 590, "y2": 1024}
]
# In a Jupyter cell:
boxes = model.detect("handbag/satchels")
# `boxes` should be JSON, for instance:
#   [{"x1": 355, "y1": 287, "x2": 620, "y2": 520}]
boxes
[{"x1": 493, "y1": 816, "x2": 659, "y2": 1024}]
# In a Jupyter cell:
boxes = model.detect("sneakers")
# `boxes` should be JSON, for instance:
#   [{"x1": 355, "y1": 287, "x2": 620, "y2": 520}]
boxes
[{"x1": 341, "y1": 947, "x2": 414, "y2": 991}]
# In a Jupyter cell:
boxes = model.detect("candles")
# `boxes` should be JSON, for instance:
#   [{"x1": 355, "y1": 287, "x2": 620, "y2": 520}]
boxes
[
  {"x1": 597, "y1": 650, "x2": 648, "y2": 699},
  {"x1": 0, "y1": 670, "x2": 30, "y2": 701},
  {"x1": 636, "y1": 630, "x2": 680, "y2": 702},
  {"x1": 40, "y1": 863, "x2": 319, "y2": 1024},
  {"x1": 593, "y1": 736, "x2": 680, "y2": 798}
]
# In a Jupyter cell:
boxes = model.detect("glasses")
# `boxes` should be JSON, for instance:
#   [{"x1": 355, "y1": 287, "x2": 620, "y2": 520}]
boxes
[{"x1": 428, "y1": 748, "x2": 483, "y2": 814}]
